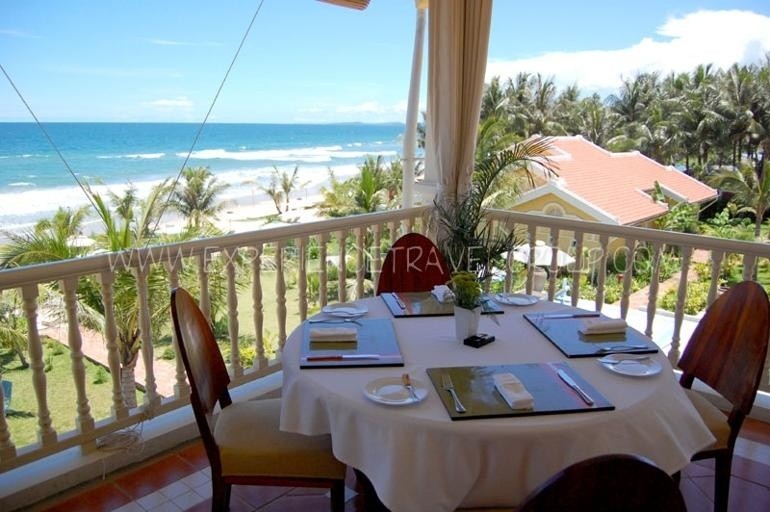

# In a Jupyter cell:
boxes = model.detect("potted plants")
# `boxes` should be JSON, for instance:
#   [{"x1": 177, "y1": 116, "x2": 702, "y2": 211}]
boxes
[{"x1": 447, "y1": 270, "x2": 482, "y2": 341}]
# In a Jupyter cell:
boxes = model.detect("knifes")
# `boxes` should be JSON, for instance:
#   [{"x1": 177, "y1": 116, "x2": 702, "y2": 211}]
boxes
[
  {"x1": 556, "y1": 369, "x2": 595, "y2": 405},
  {"x1": 391, "y1": 292, "x2": 406, "y2": 309},
  {"x1": 545, "y1": 313, "x2": 600, "y2": 318},
  {"x1": 306, "y1": 354, "x2": 379, "y2": 361}
]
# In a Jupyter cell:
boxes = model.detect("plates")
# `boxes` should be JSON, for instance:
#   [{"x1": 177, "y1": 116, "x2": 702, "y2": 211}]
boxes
[
  {"x1": 322, "y1": 301, "x2": 368, "y2": 317},
  {"x1": 493, "y1": 293, "x2": 538, "y2": 306},
  {"x1": 362, "y1": 376, "x2": 431, "y2": 407},
  {"x1": 601, "y1": 355, "x2": 662, "y2": 377}
]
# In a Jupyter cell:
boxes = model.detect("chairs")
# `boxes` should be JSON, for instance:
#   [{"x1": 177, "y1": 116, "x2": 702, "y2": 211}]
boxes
[
  {"x1": 673, "y1": 280, "x2": 766, "y2": 512},
  {"x1": 171, "y1": 286, "x2": 347, "y2": 511},
  {"x1": 514, "y1": 453, "x2": 686, "y2": 511},
  {"x1": 374, "y1": 232, "x2": 455, "y2": 296}
]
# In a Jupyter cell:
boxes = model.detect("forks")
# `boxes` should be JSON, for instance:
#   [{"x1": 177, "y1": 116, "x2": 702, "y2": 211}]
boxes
[
  {"x1": 308, "y1": 314, "x2": 363, "y2": 324},
  {"x1": 442, "y1": 375, "x2": 467, "y2": 412},
  {"x1": 401, "y1": 373, "x2": 420, "y2": 402},
  {"x1": 592, "y1": 343, "x2": 649, "y2": 351}
]
